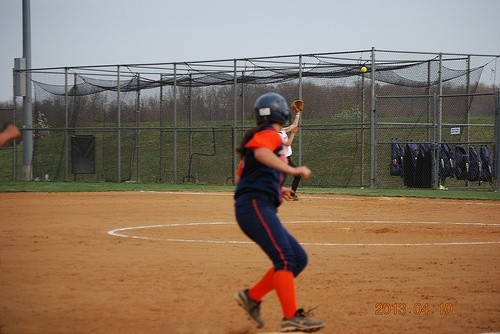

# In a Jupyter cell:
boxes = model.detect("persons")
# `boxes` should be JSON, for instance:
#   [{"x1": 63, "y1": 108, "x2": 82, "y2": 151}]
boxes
[
  {"x1": 233, "y1": 92, "x2": 325, "y2": 333},
  {"x1": 0, "y1": 124, "x2": 21, "y2": 147}
]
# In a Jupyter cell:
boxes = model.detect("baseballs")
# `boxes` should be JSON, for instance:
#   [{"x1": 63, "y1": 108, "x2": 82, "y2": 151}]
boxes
[{"x1": 361, "y1": 67, "x2": 367, "y2": 72}]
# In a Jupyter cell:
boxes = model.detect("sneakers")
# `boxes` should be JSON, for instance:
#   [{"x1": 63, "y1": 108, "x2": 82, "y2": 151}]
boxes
[
  {"x1": 235, "y1": 289, "x2": 265, "y2": 328},
  {"x1": 292, "y1": 195, "x2": 299, "y2": 201},
  {"x1": 280, "y1": 306, "x2": 325, "y2": 332}
]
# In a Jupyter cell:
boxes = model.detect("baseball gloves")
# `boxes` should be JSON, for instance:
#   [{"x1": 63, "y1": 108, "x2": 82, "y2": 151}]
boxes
[{"x1": 291, "y1": 100, "x2": 303, "y2": 112}]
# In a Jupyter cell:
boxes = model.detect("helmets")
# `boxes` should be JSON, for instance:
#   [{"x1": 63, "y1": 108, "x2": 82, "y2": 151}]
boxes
[{"x1": 252, "y1": 92, "x2": 289, "y2": 123}]
